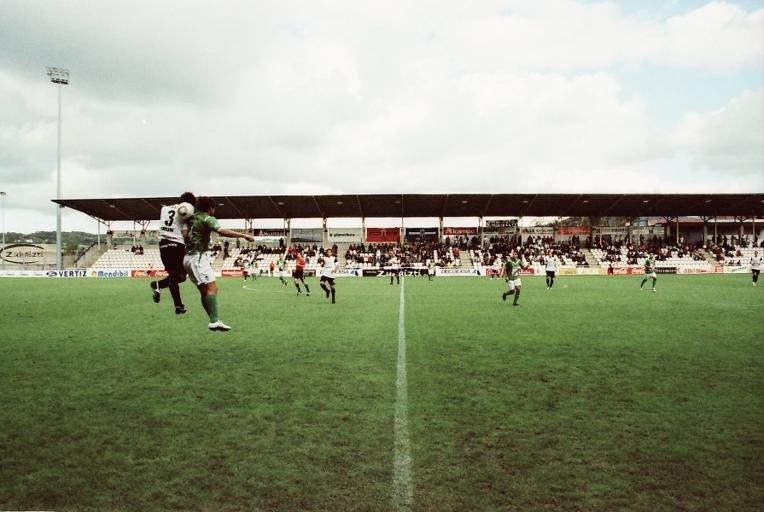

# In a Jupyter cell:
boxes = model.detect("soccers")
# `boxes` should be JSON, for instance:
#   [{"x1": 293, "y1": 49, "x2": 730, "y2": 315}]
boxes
[{"x1": 176, "y1": 202, "x2": 195, "y2": 219}]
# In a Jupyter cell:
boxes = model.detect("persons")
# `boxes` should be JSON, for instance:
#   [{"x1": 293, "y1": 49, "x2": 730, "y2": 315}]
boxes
[
  {"x1": 181, "y1": 195, "x2": 254, "y2": 332},
  {"x1": 208, "y1": 230, "x2": 763, "y2": 280},
  {"x1": 277, "y1": 253, "x2": 311, "y2": 297},
  {"x1": 130, "y1": 244, "x2": 143, "y2": 255},
  {"x1": 545, "y1": 252, "x2": 556, "y2": 289},
  {"x1": 503, "y1": 251, "x2": 530, "y2": 305},
  {"x1": 149, "y1": 191, "x2": 195, "y2": 315},
  {"x1": 320, "y1": 249, "x2": 339, "y2": 304},
  {"x1": 390, "y1": 254, "x2": 400, "y2": 284},
  {"x1": 750, "y1": 251, "x2": 762, "y2": 285},
  {"x1": 640, "y1": 253, "x2": 657, "y2": 291}
]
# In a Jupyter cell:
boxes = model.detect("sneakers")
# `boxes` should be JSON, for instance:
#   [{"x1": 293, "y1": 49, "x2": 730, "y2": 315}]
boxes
[
  {"x1": 208, "y1": 321, "x2": 231, "y2": 331},
  {"x1": 503, "y1": 292, "x2": 506, "y2": 300},
  {"x1": 513, "y1": 302, "x2": 519, "y2": 305},
  {"x1": 296, "y1": 290, "x2": 336, "y2": 304},
  {"x1": 176, "y1": 307, "x2": 186, "y2": 314},
  {"x1": 150, "y1": 281, "x2": 160, "y2": 303}
]
